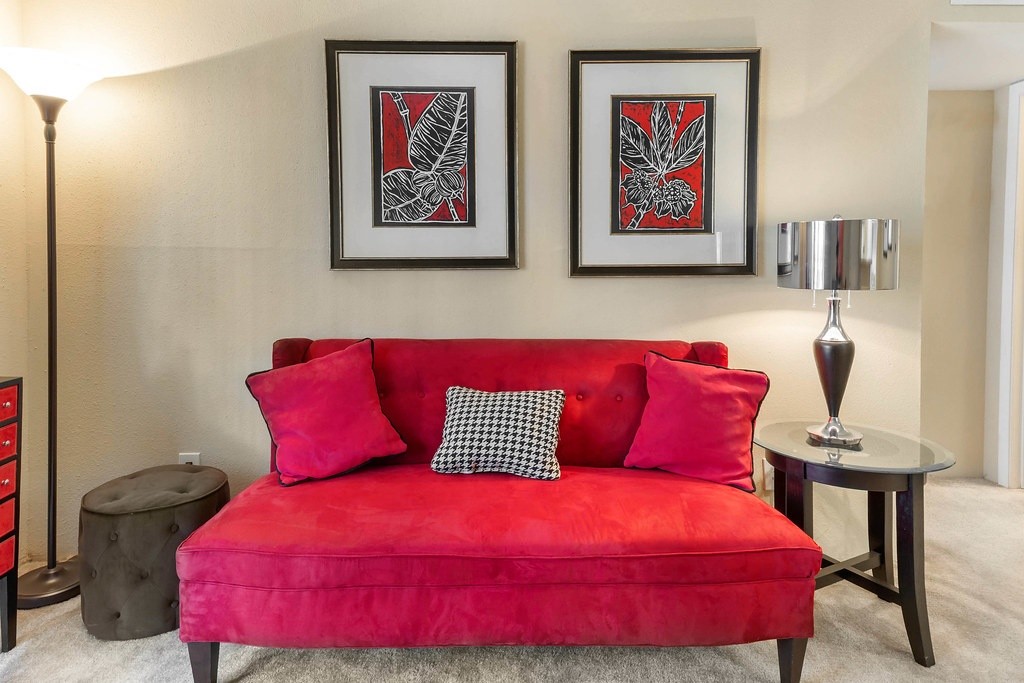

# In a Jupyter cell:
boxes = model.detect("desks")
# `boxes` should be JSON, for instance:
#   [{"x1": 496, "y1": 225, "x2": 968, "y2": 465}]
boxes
[{"x1": 751, "y1": 424, "x2": 959, "y2": 683}]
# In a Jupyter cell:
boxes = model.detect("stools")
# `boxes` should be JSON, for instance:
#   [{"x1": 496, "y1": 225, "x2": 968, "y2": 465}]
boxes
[{"x1": 77, "y1": 465, "x2": 231, "y2": 641}]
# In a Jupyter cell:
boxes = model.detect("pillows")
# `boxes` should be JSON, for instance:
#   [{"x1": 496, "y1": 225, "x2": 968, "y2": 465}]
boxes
[
  {"x1": 245, "y1": 338, "x2": 409, "y2": 488},
  {"x1": 430, "y1": 386, "x2": 566, "y2": 480},
  {"x1": 622, "y1": 351, "x2": 771, "y2": 493}
]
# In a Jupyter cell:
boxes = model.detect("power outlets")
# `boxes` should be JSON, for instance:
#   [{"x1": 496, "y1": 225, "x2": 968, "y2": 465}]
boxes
[
  {"x1": 762, "y1": 458, "x2": 775, "y2": 491},
  {"x1": 178, "y1": 453, "x2": 202, "y2": 466}
]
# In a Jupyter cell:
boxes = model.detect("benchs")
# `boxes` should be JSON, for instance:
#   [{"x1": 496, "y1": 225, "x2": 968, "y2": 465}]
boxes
[{"x1": 174, "y1": 336, "x2": 822, "y2": 683}]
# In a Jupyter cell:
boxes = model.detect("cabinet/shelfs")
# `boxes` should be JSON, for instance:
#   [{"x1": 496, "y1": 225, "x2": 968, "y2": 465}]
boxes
[{"x1": 0, "y1": 375, "x2": 24, "y2": 653}]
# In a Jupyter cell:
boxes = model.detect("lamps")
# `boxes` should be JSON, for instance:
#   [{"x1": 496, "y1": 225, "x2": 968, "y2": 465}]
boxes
[
  {"x1": 776, "y1": 216, "x2": 901, "y2": 446},
  {"x1": 0, "y1": 46, "x2": 103, "y2": 614}
]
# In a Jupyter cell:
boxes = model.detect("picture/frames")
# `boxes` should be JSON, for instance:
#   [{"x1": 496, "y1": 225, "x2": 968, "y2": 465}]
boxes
[
  {"x1": 569, "y1": 48, "x2": 764, "y2": 279},
  {"x1": 324, "y1": 39, "x2": 522, "y2": 271}
]
